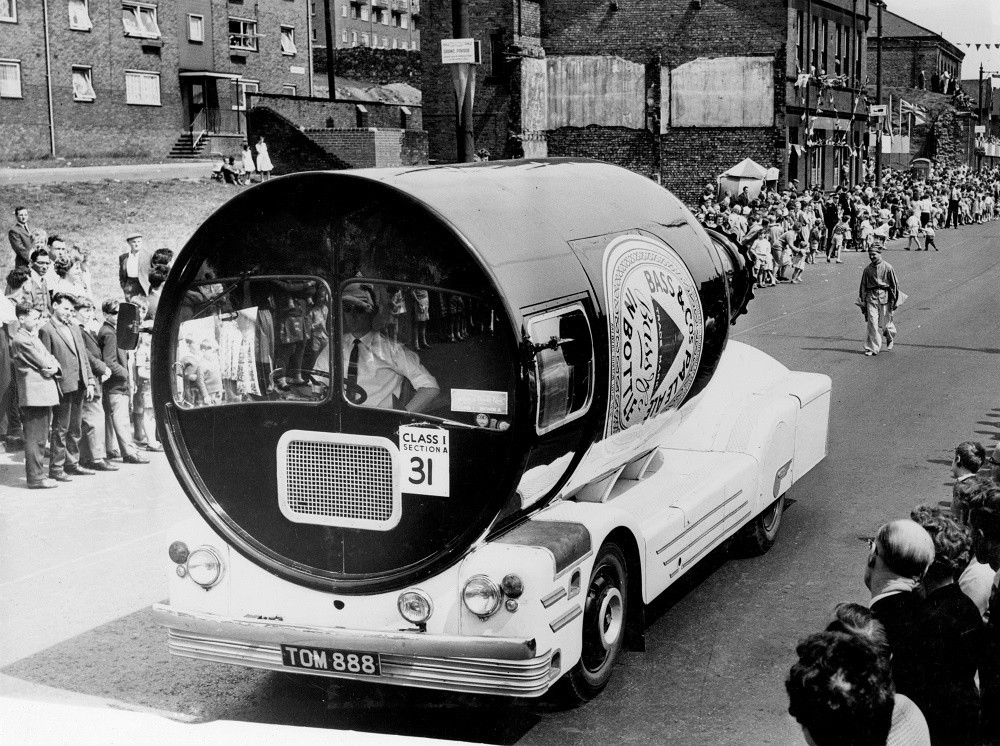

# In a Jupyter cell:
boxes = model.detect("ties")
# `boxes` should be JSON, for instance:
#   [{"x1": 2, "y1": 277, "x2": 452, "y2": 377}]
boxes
[{"x1": 346, "y1": 339, "x2": 361, "y2": 403}]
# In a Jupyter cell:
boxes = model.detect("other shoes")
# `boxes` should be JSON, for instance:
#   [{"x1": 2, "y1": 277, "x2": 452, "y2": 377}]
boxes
[
  {"x1": 135, "y1": 442, "x2": 166, "y2": 452},
  {"x1": 49, "y1": 472, "x2": 73, "y2": 481},
  {"x1": 67, "y1": 469, "x2": 96, "y2": 475},
  {"x1": 294, "y1": 380, "x2": 310, "y2": 386},
  {"x1": 27, "y1": 477, "x2": 58, "y2": 488},
  {"x1": 277, "y1": 383, "x2": 291, "y2": 391}
]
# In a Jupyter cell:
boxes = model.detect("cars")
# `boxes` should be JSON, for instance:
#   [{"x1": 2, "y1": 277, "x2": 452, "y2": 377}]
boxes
[{"x1": 138, "y1": 150, "x2": 838, "y2": 703}]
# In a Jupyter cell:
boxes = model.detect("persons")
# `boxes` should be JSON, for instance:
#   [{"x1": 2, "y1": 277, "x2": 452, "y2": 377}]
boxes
[
  {"x1": 784, "y1": 441, "x2": 999, "y2": 746},
  {"x1": 688, "y1": 162, "x2": 1000, "y2": 356},
  {"x1": 181, "y1": 265, "x2": 477, "y2": 390},
  {"x1": 1, "y1": 139, "x2": 273, "y2": 486},
  {"x1": 269, "y1": 281, "x2": 441, "y2": 413}
]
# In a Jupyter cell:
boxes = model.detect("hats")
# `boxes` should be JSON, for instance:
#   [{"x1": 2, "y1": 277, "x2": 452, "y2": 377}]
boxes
[
  {"x1": 342, "y1": 283, "x2": 378, "y2": 316},
  {"x1": 127, "y1": 233, "x2": 142, "y2": 242},
  {"x1": 868, "y1": 242, "x2": 882, "y2": 253}
]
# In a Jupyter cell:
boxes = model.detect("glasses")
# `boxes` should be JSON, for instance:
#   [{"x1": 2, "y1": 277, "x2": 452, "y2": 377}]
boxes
[
  {"x1": 988, "y1": 457, "x2": 999, "y2": 465},
  {"x1": 108, "y1": 311, "x2": 119, "y2": 315},
  {"x1": 343, "y1": 304, "x2": 364, "y2": 314}
]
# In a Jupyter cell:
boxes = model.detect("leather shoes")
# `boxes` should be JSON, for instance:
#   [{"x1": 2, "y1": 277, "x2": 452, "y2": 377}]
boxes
[
  {"x1": 107, "y1": 449, "x2": 151, "y2": 464},
  {"x1": 80, "y1": 456, "x2": 120, "y2": 471}
]
